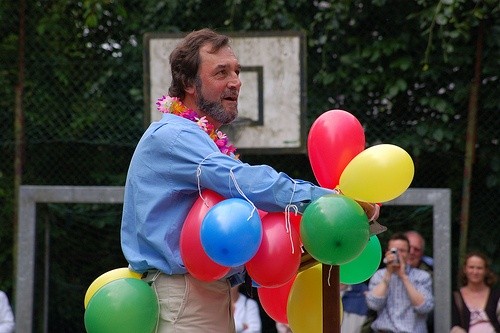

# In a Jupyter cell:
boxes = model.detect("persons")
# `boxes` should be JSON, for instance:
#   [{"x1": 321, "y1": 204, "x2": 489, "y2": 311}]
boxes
[
  {"x1": 367, "y1": 233, "x2": 434, "y2": 333},
  {"x1": 230, "y1": 285, "x2": 261, "y2": 333},
  {"x1": 0, "y1": 291, "x2": 15, "y2": 333},
  {"x1": 453, "y1": 252, "x2": 500, "y2": 333},
  {"x1": 404, "y1": 230, "x2": 433, "y2": 277},
  {"x1": 121, "y1": 29, "x2": 376, "y2": 333}
]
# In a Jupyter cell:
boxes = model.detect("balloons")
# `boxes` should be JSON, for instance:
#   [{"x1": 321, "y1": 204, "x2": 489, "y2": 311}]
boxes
[
  {"x1": 179, "y1": 189, "x2": 230, "y2": 282},
  {"x1": 340, "y1": 235, "x2": 382, "y2": 285},
  {"x1": 84, "y1": 268, "x2": 142, "y2": 309},
  {"x1": 201, "y1": 198, "x2": 263, "y2": 267},
  {"x1": 300, "y1": 194, "x2": 370, "y2": 266},
  {"x1": 84, "y1": 277, "x2": 158, "y2": 333},
  {"x1": 245, "y1": 211, "x2": 302, "y2": 288},
  {"x1": 287, "y1": 263, "x2": 343, "y2": 333},
  {"x1": 339, "y1": 144, "x2": 414, "y2": 204},
  {"x1": 257, "y1": 272, "x2": 297, "y2": 324},
  {"x1": 308, "y1": 110, "x2": 366, "y2": 189}
]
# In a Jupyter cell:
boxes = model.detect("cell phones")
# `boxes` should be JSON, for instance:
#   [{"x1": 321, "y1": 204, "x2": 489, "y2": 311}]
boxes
[{"x1": 227, "y1": 272, "x2": 247, "y2": 289}]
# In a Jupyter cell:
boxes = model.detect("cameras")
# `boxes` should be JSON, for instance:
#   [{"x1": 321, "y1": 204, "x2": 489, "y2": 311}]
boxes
[{"x1": 391, "y1": 247, "x2": 399, "y2": 265}]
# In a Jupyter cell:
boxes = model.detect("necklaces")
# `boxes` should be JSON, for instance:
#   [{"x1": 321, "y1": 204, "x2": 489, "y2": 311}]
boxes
[{"x1": 157, "y1": 96, "x2": 237, "y2": 156}]
